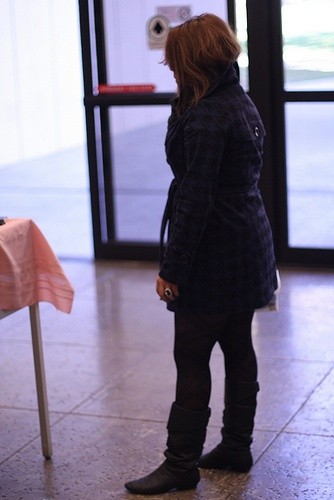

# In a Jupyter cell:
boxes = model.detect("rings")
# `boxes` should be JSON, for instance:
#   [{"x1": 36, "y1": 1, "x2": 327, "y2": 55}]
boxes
[{"x1": 164, "y1": 288, "x2": 173, "y2": 298}]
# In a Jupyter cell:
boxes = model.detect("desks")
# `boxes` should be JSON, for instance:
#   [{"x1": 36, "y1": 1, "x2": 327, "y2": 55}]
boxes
[{"x1": 0, "y1": 217, "x2": 74, "y2": 458}]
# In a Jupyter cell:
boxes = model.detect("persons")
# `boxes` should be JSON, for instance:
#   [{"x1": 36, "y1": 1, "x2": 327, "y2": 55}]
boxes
[{"x1": 123, "y1": 13, "x2": 279, "y2": 494}]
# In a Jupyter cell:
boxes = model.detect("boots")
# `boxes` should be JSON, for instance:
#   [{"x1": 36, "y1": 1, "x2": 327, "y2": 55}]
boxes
[
  {"x1": 199, "y1": 405, "x2": 260, "y2": 472},
  {"x1": 125, "y1": 403, "x2": 211, "y2": 495}
]
